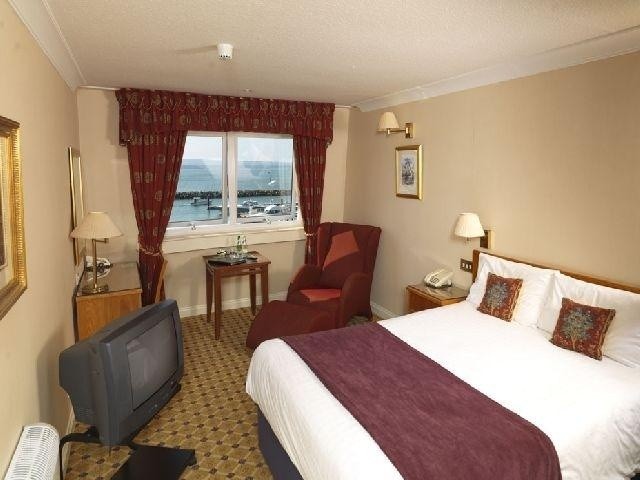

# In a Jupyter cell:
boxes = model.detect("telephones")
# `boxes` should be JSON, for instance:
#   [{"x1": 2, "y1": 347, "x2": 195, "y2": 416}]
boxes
[{"x1": 423, "y1": 268, "x2": 454, "y2": 288}]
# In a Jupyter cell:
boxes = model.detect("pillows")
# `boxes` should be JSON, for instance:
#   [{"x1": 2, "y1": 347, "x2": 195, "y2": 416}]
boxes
[
  {"x1": 475, "y1": 272, "x2": 525, "y2": 324},
  {"x1": 537, "y1": 268, "x2": 639, "y2": 372},
  {"x1": 547, "y1": 297, "x2": 616, "y2": 364},
  {"x1": 466, "y1": 252, "x2": 558, "y2": 327}
]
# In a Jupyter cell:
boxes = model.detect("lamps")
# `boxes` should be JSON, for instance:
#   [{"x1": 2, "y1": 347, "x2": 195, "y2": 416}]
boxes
[
  {"x1": 378, "y1": 110, "x2": 413, "y2": 139},
  {"x1": 454, "y1": 211, "x2": 492, "y2": 248},
  {"x1": 69, "y1": 212, "x2": 123, "y2": 295}
]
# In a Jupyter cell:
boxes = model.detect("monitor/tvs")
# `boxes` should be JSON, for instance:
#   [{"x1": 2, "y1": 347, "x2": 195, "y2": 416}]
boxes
[{"x1": 59, "y1": 299, "x2": 184, "y2": 446}]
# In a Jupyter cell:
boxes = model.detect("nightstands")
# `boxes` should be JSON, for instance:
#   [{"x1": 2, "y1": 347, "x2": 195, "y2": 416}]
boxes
[{"x1": 403, "y1": 283, "x2": 470, "y2": 316}]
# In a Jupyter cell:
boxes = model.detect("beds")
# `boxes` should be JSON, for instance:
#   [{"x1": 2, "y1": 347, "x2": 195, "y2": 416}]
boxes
[{"x1": 239, "y1": 248, "x2": 639, "y2": 480}]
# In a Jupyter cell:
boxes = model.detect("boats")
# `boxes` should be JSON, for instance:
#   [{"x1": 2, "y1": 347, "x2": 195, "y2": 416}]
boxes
[
  {"x1": 268, "y1": 178, "x2": 276, "y2": 187},
  {"x1": 191, "y1": 194, "x2": 299, "y2": 222}
]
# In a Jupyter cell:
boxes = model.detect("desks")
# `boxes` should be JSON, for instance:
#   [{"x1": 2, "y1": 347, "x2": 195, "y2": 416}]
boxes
[
  {"x1": 74, "y1": 260, "x2": 144, "y2": 341},
  {"x1": 203, "y1": 250, "x2": 273, "y2": 342}
]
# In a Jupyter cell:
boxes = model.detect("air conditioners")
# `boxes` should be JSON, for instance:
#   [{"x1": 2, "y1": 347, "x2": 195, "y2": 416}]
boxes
[{"x1": 2, "y1": 423, "x2": 61, "y2": 480}]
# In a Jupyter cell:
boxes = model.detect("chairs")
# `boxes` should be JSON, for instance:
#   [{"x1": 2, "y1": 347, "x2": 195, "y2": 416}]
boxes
[
  {"x1": 245, "y1": 221, "x2": 383, "y2": 352},
  {"x1": 144, "y1": 250, "x2": 169, "y2": 306}
]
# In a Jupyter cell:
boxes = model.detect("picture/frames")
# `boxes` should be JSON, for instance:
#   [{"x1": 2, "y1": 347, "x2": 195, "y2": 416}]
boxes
[
  {"x1": 67, "y1": 145, "x2": 89, "y2": 268},
  {"x1": 1, "y1": 114, "x2": 29, "y2": 325},
  {"x1": 394, "y1": 143, "x2": 423, "y2": 201}
]
